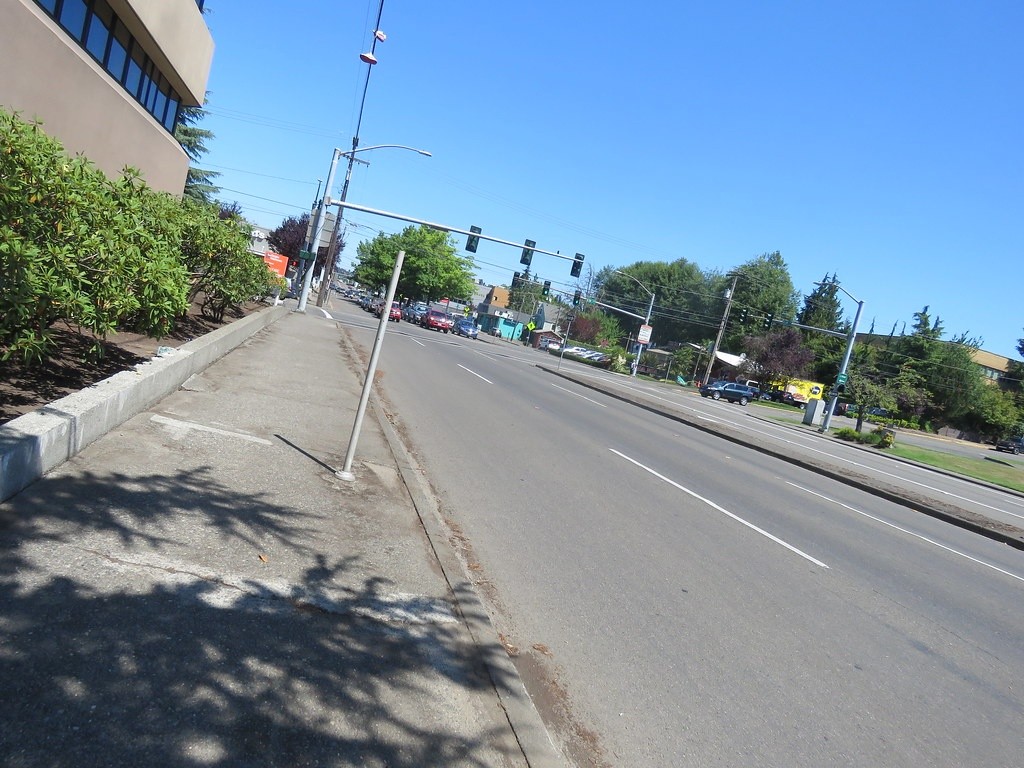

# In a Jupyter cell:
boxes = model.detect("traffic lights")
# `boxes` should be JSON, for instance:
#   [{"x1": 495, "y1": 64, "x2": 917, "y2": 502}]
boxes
[
  {"x1": 737, "y1": 308, "x2": 749, "y2": 323},
  {"x1": 573, "y1": 290, "x2": 582, "y2": 306},
  {"x1": 543, "y1": 280, "x2": 551, "y2": 296},
  {"x1": 512, "y1": 272, "x2": 520, "y2": 287},
  {"x1": 762, "y1": 313, "x2": 774, "y2": 331}
]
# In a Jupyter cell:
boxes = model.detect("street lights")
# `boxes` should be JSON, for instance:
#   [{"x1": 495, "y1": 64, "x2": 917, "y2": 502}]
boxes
[
  {"x1": 291, "y1": 144, "x2": 433, "y2": 313},
  {"x1": 614, "y1": 270, "x2": 656, "y2": 377},
  {"x1": 813, "y1": 280, "x2": 867, "y2": 431}
]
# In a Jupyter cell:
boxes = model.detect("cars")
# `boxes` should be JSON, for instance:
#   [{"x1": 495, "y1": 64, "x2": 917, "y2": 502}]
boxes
[
  {"x1": 563, "y1": 346, "x2": 612, "y2": 365},
  {"x1": 771, "y1": 390, "x2": 795, "y2": 406},
  {"x1": 329, "y1": 281, "x2": 479, "y2": 340},
  {"x1": 750, "y1": 388, "x2": 772, "y2": 402},
  {"x1": 995, "y1": 435, "x2": 1024, "y2": 455},
  {"x1": 822, "y1": 400, "x2": 895, "y2": 424}
]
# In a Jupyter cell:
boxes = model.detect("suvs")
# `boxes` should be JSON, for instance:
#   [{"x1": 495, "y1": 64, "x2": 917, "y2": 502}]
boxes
[{"x1": 698, "y1": 380, "x2": 754, "y2": 406}]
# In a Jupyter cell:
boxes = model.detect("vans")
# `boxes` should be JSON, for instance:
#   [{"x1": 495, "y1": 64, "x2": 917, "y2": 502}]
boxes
[{"x1": 738, "y1": 379, "x2": 760, "y2": 389}]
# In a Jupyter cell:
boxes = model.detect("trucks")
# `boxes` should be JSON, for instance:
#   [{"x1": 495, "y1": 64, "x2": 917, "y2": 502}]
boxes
[{"x1": 763, "y1": 373, "x2": 825, "y2": 410}]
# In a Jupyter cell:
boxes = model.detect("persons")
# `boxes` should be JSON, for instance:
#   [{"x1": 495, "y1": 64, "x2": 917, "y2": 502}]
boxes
[
  {"x1": 844, "y1": 404, "x2": 848, "y2": 411},
  {"x1": 628, "y1": 359, "x2": 635, "y2": 378}
]
